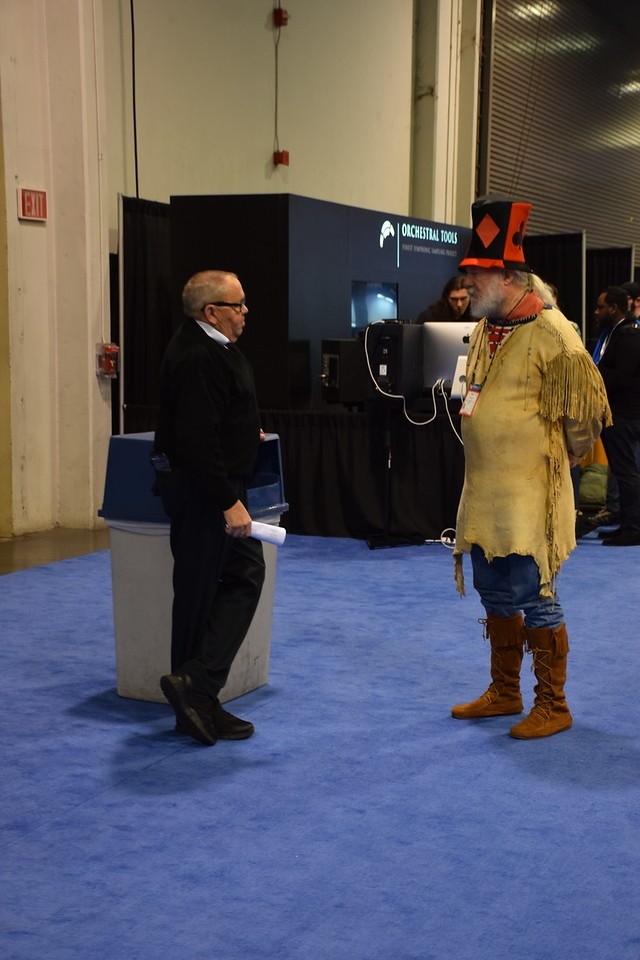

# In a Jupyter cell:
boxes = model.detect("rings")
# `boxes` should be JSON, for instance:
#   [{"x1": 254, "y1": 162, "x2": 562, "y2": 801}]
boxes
[{"x1": 241, "y1": 532, "x2": 246, "y2": 535}]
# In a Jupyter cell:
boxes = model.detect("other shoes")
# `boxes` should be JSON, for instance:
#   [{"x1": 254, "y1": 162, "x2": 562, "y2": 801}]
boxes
[{"x1": 598, "y1": 522, "x2": 640, "y2": 546}]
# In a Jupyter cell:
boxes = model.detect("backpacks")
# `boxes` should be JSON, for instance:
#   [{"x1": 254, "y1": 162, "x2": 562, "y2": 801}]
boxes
[{"x1": 577, "y1": 463, "x2": 607, "y2": 505}]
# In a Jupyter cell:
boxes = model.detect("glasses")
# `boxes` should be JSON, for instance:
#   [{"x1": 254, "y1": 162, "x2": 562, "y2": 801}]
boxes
[{"x1": 202, "y1": 299, "x2": 247, "y2": 312}]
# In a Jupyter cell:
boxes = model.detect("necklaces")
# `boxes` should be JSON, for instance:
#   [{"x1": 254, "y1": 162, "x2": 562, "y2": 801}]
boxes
[{"x1": 484, "y1": 322, "x2": 527, "y2": 373}]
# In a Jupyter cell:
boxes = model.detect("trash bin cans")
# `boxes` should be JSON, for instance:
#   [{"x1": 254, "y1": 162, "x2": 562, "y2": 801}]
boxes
[{"x1": 95, "y1": 431, "x2": 288, "y2": 706}]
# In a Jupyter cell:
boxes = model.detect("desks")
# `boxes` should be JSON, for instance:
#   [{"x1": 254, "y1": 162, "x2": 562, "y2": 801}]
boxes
[{"x1": 257, "y1": 409, "x2": 465, "y2": 541}]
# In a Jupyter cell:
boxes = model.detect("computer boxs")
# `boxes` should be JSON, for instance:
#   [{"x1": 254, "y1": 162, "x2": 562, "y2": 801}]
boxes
[{"x1": 368, "y1": 320, "x2": 423, "y2": 400}]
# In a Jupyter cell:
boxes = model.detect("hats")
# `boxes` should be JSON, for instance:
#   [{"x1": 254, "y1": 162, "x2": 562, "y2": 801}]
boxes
[
  {"x1": 458, "y1": 199, "x2": 533, "y2": 273},
  {"x1": 619, "y1": 282, "x2": 640, "y2": 300}
]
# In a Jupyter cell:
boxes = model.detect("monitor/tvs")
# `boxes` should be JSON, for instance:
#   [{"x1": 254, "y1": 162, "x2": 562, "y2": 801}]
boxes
[{"x1": 423, "y1": 321, "x2": 479, "y2": 399}]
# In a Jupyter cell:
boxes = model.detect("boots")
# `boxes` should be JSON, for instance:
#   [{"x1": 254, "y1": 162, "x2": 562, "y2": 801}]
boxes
[
  {"x1": 451, "y1": 612, "x2": 525, "y2": 720},
  {"x1": 509, "y1": 623, "x2": 572, "y2": 739}
]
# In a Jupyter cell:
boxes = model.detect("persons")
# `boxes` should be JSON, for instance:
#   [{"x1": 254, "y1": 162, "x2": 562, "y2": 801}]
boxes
[
  {"x1": 152, "y1": 271, "x2": 266, "y2": 747},
  {"x1": 451, "y1": 197, "x2": 613, "y2": 738},
  {"x1": 593, "y1": 282, "x2": 640, "y2": 547},
  {"x1": 416, "y1": 274, "x2": 583, "y2": 522}
]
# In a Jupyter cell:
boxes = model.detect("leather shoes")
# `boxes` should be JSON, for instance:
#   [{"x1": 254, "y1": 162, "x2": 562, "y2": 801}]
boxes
[
  {"x1": 174, "y1": 701, "x2": 256, "y2": 742},
  {"x1": 160, "y1": 671, "x2": 220, "y2": 746}
]
